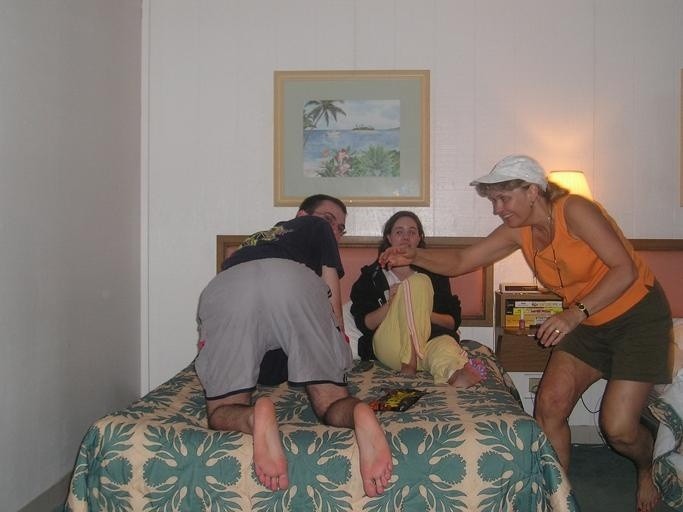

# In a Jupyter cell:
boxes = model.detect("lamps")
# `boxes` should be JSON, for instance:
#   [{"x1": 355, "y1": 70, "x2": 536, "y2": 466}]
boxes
[{"x1": 546, "y1": 171, "x2": 592, "y2": 200}]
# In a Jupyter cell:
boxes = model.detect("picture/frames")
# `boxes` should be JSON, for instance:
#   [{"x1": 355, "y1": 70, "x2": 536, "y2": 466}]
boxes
[{"x1": 273, "y1": 70, "x2": 429, "y2": 208}]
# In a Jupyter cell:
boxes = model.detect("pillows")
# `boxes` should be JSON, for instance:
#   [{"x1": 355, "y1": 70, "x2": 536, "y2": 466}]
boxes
[{"x1": 341, "y1": 301, "x2": 363, "y2": 360}]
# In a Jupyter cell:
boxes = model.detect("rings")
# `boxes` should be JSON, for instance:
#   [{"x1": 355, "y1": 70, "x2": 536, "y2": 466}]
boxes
[{"x1": 554, "y1": 329, "x2": 560, "y2": 335}]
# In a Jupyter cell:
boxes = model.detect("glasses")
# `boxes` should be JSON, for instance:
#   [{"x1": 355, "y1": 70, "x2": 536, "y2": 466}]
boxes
[{"x1": 313, "y1": 210, "x2": 345, "y2": 234}]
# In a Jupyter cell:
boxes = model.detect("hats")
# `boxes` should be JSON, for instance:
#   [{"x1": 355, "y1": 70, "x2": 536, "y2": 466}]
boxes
[{"x1": 469, "y1": 156, "x2": 549, "y2": 192}]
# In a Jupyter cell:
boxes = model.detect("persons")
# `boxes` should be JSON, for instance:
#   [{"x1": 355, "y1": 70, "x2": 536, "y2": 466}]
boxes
[
  {"x1": 350, "y1": 211, "x2": 487, "y2": 388},
  {"x1": 379, "y1": 154, "x2": 672, "y2": 512},
  {"x1": 195, "y1": 194, "x2": 394, "y2": 498}
]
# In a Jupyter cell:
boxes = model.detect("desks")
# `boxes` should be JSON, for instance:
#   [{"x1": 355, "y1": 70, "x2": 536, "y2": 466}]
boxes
[{"x1": 494, "y1": 291, "x2": 566, "y2": 372}]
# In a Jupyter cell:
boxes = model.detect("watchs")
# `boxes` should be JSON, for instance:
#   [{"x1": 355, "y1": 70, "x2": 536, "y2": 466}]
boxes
[{"x1": 575, "y1": 302, "x2": 590, "y2": 318}]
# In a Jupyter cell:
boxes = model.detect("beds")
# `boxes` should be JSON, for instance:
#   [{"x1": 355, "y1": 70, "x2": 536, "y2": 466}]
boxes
[
  {"x1": 64, "y1": 235, "x2": 576, "y2": 511},
  {"x1": 627, "y1": 238, "x2": 681, "y2": 511}
]
